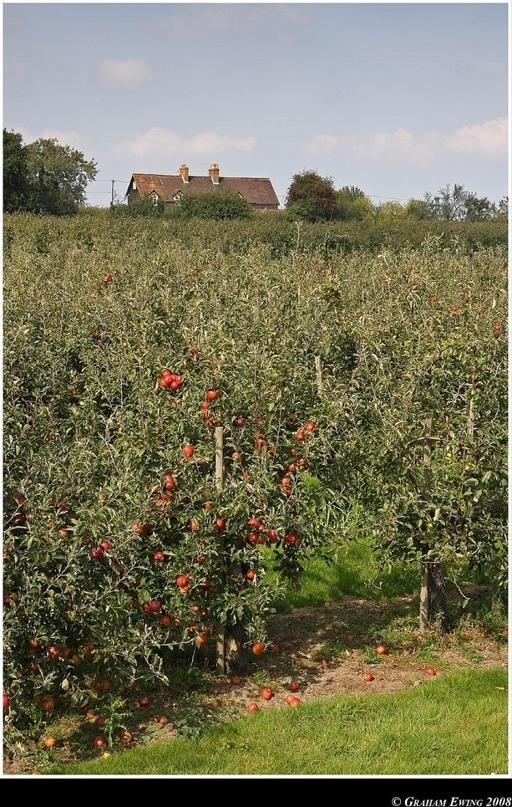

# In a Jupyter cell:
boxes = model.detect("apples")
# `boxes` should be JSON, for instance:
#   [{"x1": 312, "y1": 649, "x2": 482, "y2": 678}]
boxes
[{"x1": 3, "y1": 370, "x2": 436, "y2": 758}]
